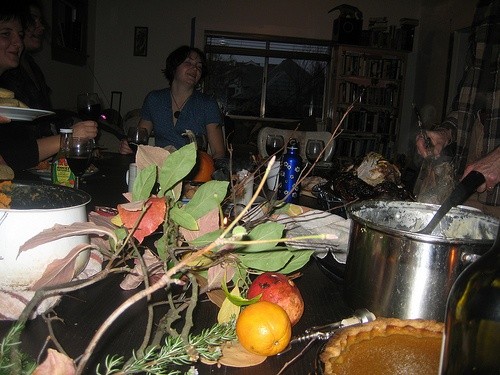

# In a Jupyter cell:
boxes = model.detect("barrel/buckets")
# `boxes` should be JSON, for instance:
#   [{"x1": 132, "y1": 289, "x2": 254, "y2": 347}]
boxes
[{"x1": 342, "y1": 198, "x2": 500, "y2": 319}]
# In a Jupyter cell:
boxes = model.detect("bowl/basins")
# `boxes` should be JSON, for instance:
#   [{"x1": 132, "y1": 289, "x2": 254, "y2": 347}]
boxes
[{"x1": 312, "y1": 180, "x2": 377, "y2": 214}]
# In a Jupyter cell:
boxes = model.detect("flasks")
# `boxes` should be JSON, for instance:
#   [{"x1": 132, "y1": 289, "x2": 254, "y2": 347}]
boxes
[{"x1": 276, "y1": 137, "x2": 302, "y2": 204}]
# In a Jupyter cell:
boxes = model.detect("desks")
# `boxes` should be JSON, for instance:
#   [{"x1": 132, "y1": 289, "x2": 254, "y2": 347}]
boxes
[{"x1": 0, "y1": 154, "x2": 345, "y2": 375}]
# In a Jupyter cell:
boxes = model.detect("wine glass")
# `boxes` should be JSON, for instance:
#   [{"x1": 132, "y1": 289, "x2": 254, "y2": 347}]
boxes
[
  {"x1": 305, "y1": 140, "x2": 325, "y2": 177},
  {"x1": 65, "y1": 137, "x2": 95, "y2": 189},
  {"x1": 79, "y1": 92, "x2": 105, "y2": 147}
]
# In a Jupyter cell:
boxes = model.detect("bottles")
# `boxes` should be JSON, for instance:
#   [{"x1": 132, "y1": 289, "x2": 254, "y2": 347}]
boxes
[
  {"x1": 438, "y1": 223, "x2": 500, "y2": 375},
  {"x1": 51, "y1": 128, "x2": 77, "y2": 188}
]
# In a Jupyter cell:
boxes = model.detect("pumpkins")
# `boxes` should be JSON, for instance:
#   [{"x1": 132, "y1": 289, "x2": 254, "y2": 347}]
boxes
[{"x1": 183, "y1": 129, "x2": 213, "y2": 182}]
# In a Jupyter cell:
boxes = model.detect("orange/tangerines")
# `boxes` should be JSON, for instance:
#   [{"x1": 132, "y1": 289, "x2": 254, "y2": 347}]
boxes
[{"x1": 237, "y1": 301, "x2": 291, "y2": 356}]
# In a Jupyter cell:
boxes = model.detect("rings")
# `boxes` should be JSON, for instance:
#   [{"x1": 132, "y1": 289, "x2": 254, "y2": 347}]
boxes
[{"x1": 487, "y1": 185, "x2": 494, "y2": 189}]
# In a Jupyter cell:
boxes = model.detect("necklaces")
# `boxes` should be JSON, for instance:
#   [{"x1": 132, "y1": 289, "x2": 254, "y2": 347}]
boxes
[{"x1": 171, "y1": 90, "x2": 191, "y2": 119}]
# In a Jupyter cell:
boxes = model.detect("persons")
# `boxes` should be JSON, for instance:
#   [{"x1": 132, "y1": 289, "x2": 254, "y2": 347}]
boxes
[
  {"x1": 417, "y1": 0, "x2": 500, "y2": 206},
  {"x1": 118, "y1": 45, "x2": 226, "y2": 169},
  {"x1": 0, "y1": 0, "x2": 98, "y2": 171}
]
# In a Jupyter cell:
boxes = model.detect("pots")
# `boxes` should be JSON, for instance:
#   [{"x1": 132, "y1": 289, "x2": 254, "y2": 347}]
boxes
[{"x1": 0, "y1": 182, "x2": 91, "y2": 291}]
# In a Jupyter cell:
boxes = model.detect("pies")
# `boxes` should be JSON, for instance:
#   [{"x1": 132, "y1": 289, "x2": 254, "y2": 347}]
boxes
[{"x1": 319, "y1": 317, "x2": 446, "y2": 375}]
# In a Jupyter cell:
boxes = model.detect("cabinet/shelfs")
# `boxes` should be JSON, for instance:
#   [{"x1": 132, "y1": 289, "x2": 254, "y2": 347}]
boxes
[{"x1": 324, "y1": 45, "x2": 407, "y2": 168}]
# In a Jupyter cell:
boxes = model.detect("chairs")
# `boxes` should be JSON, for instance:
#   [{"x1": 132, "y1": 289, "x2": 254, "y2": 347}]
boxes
[{"x1": 257, "y1": 127, "x2": 335, "y2": 165}]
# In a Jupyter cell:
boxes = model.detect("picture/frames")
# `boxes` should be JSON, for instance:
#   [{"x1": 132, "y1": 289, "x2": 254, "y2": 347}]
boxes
[{"x1": 133, "y1": 26, "x2": 148, "y2": 57}]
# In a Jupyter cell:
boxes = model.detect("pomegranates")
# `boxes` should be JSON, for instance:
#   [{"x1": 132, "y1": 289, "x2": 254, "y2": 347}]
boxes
[{"x1": 246, "y1": 271, "x2": 304, "y2": 327}]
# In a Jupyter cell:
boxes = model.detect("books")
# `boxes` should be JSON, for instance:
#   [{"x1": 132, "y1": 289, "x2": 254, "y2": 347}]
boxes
[
  {"x1": 334, "y1": 137, "x2": 394, "y2": 158},
  {"x1": 340, "y1": 55, "x2": 402, "y2": 80},
  {"x1": 338, "y1": 83, "x2": 397, "y2": 106},
  {"x1": 336, "y1": 108, "x2": 398, "y2": 132}
]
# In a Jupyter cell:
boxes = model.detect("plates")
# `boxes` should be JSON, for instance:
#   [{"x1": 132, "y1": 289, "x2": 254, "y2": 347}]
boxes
[
  {"x1": 28, "y1": 162, "x2": 98, "y2": 181},
  {"x1": 0, "y1": 106, "x2": 55, "y2": 121}
]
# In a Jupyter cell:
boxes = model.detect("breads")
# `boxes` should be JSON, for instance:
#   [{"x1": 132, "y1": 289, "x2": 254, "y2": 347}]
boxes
[{"x1": 0, "y1": 86, "x2": 29, "y2": 108}]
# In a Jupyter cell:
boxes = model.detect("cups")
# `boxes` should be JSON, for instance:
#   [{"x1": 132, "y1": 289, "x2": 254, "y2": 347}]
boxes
[
  {"x1": 265, "y1": 135, "x2": 285, "y2": 160},
  {"x1": 233, "y1": 171, "x2": 254, "y2": 215},
  {"x1": 128, "y1": 162, "x2": 137, "y2": 194},
  {"x1": 266, "y1": 161, "x2": 280, "y2": 191},
  {"x1": 127, "y1": 126, "x2": 148, "y2": 155}
]
